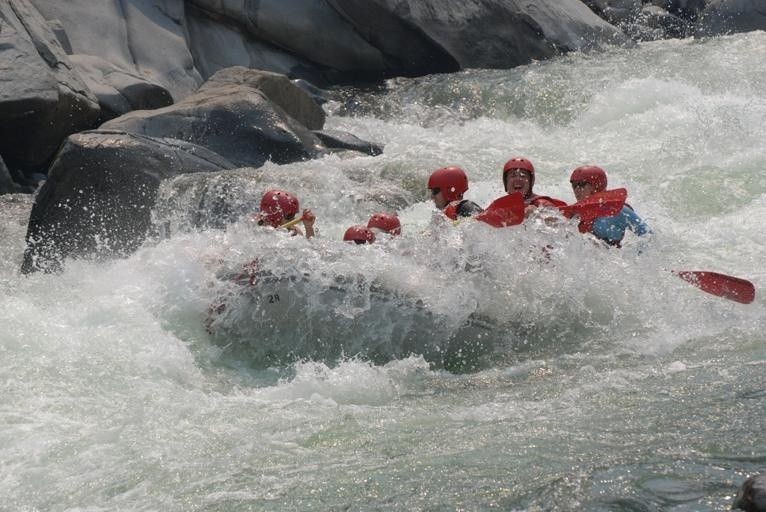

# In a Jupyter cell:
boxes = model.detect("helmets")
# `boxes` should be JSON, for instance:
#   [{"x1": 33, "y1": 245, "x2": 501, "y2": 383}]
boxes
[
  {"x1": 259, "y1": 190, "x2": 300, "y2": 223},
  {"x1": 569, "y1": 166, "x2": 607, "y2": 191},
  {"x1": 502, "y1": 158, "x2": 536, "y2": 186},
  {"x1": 427, "y1": 167, "x2": 469, "y2": 201},
  {"x1": 343, "y1": 224, "x2": 375, "y2": 246}
]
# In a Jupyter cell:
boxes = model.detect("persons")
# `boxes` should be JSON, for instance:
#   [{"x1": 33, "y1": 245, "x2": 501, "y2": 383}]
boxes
[
  {"x1": 426, "y1": 165, "x2": 481, "y2": 222},
  {"x1": 248, "y1": 189, "x2": 314, "y2": 238},
  {"x1": 502, "y1": 157, "x2": 567, "y2": 222},
  {"x1": 562, "y1": 166, "x2": 654, "y2": 247},
  {"x1": 344, "y1": 224, "x2": 373, "y2": 244}
]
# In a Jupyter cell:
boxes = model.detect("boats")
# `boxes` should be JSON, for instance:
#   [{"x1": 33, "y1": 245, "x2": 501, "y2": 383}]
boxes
[{"x1": 208, "y1": 200, "x2": 543, "y2": 377}]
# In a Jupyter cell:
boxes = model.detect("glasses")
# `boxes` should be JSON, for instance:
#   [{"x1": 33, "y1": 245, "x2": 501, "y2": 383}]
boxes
[
  {"x1": 429, "y1": 188, "x2": 440, "y2": 196},
  {"x1": 571, "y1": 182, "x2": 589, "y2": 189}
]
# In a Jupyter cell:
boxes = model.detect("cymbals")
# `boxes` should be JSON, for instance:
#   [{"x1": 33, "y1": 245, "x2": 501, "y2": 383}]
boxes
[{"x1": 555, "y1": 189, "x2": 627, "y2": 221}]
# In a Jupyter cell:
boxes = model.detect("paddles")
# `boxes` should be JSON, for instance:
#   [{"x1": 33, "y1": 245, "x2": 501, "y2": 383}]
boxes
[
  {"x1": 447, "y1": 192, "x2": 525, "y2": 228},
  {"x1": 671, "y1": 270, "x2": 755, "y2": 304}
]
